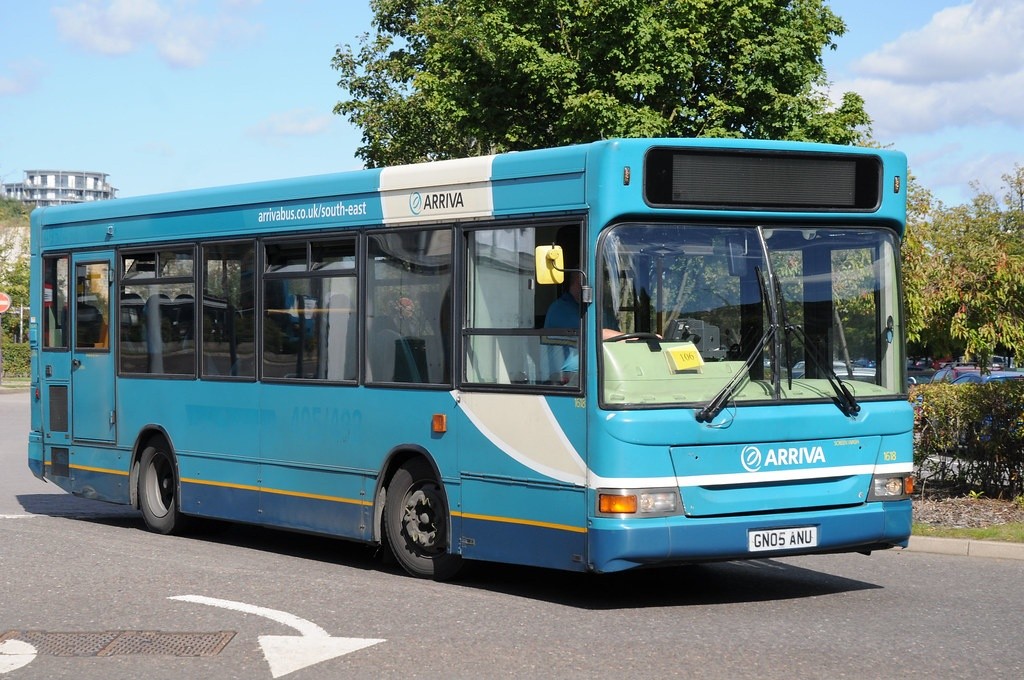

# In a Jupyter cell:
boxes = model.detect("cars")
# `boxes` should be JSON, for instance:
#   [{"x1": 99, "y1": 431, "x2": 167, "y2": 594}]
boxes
[{"x1": 780, "y1": 353, "x2": 1024, "y2": 457}]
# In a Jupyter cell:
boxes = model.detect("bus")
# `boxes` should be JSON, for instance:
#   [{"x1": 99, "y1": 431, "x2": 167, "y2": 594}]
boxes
[{"x1": 26, "y1": 135, "x2": 916, "y2": 585}]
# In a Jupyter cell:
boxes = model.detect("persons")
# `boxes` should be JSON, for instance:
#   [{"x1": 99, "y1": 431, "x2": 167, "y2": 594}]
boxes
[{"x1": 542, "y1": 263, "x2": 620, "y2": 385}]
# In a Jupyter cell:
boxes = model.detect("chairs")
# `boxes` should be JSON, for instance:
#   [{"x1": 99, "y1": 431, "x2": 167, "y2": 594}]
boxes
[{"x1": 55, "y1": 283, "x2": 453, "y2": 386}]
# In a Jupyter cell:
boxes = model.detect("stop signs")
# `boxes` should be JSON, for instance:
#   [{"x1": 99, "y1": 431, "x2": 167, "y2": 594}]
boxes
[{"x1": 0, "y1": 292, "x2": 11, "y2": 314}]
